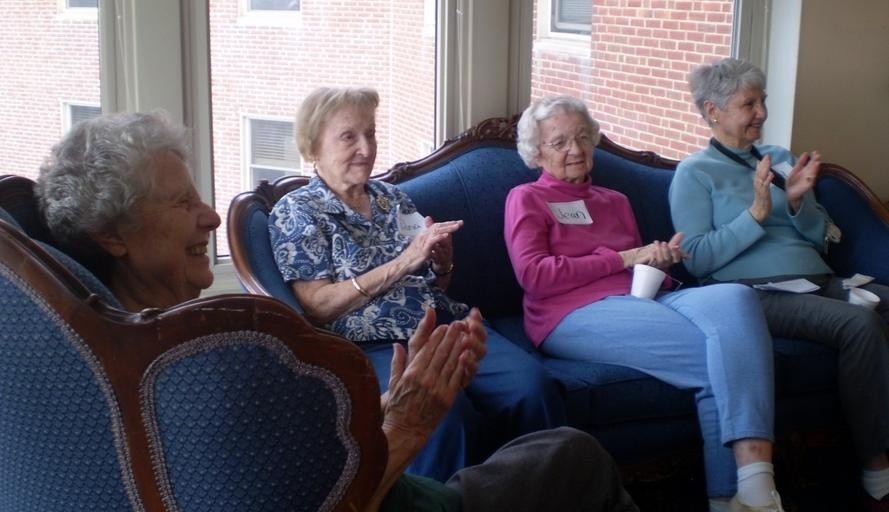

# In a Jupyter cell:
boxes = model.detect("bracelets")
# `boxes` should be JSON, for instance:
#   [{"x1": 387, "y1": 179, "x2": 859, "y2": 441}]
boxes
[{"x1": 349, "y1": 275, "x2": 373, "y2": 301}]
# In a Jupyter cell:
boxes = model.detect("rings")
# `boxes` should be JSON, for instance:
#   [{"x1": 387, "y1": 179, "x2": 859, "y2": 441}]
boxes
[{"x1": 759, "y1": 180, "x2": 764, "y2": 185}]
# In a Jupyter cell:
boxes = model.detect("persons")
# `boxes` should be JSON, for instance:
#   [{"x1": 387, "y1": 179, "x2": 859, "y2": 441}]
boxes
[
  {"x1": 502, "y1": 93, "x2": 783, "y2": 511},
  {"x1": 30, "y1": 105, "x2": 647, "y2": 510},
  {"x1": 265, "y1": 84, "x2": 565, "y2": 484},
  {"x1": 666, "y1": 56, "x2": 889, "y2": 512}
]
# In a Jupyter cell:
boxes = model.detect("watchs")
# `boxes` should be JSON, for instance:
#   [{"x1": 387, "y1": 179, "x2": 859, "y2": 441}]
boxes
[{"x1": 431, "y1": 261, "x2": 455, "y2": 275}]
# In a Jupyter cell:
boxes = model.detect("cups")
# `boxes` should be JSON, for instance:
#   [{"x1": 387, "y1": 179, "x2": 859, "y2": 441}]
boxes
[
  {"x1": 849, "y1": 287, "x2": 881, "y2": 310},
  {"x1": 631, "y1": 263, "x2": 667, "y2": 300}
]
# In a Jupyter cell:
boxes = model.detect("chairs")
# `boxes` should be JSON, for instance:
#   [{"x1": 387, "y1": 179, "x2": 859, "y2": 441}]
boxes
[{"x1": 0, "y1": 173, "x2": 390, "y2": 512}]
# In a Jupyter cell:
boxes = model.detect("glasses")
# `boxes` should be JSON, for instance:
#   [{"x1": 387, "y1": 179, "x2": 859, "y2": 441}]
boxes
[{"x1": 546, "y1": 131, "x2": 591, "y2": 152}]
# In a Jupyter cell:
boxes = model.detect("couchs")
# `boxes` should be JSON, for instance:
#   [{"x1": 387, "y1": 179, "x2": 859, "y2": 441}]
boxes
[{"x1": 225, "y1": 110, "x2": 889, "y2": 512}]
